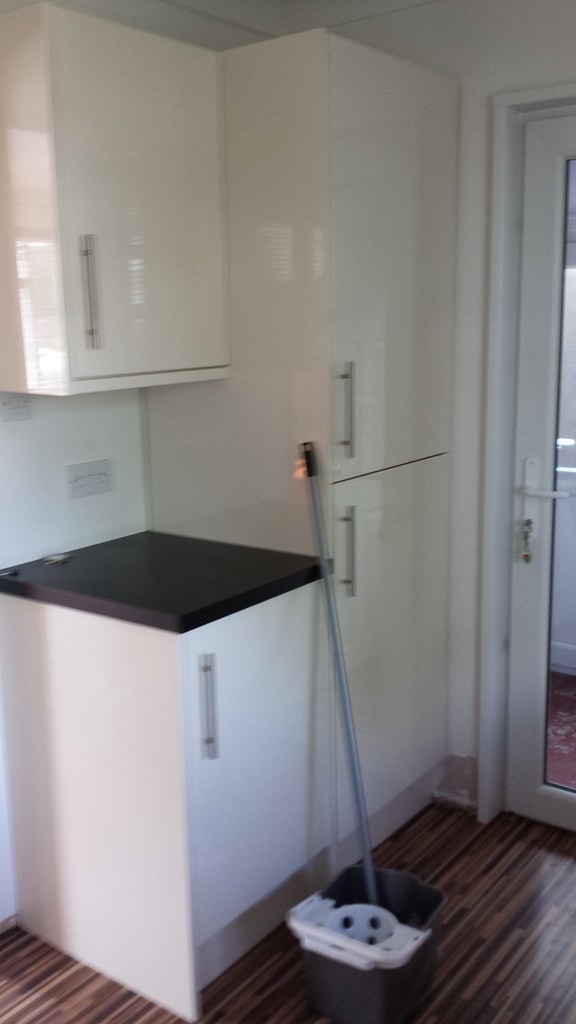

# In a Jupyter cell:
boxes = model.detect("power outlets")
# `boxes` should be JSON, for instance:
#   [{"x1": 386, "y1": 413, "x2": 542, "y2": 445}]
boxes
[{"x1": 63, "y1": 457, "x2": 113, "y2": 501}]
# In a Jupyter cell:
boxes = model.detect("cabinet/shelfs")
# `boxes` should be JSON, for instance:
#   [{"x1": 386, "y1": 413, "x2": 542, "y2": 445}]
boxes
[{"x1": 0, "y1": 0, "x2": 460, "y2": 1024}]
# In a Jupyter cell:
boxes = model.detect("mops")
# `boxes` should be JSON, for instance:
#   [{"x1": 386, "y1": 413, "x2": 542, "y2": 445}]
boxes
[{"x1": 298, "y1": 436, "x2": 380, "y2": 905}]
